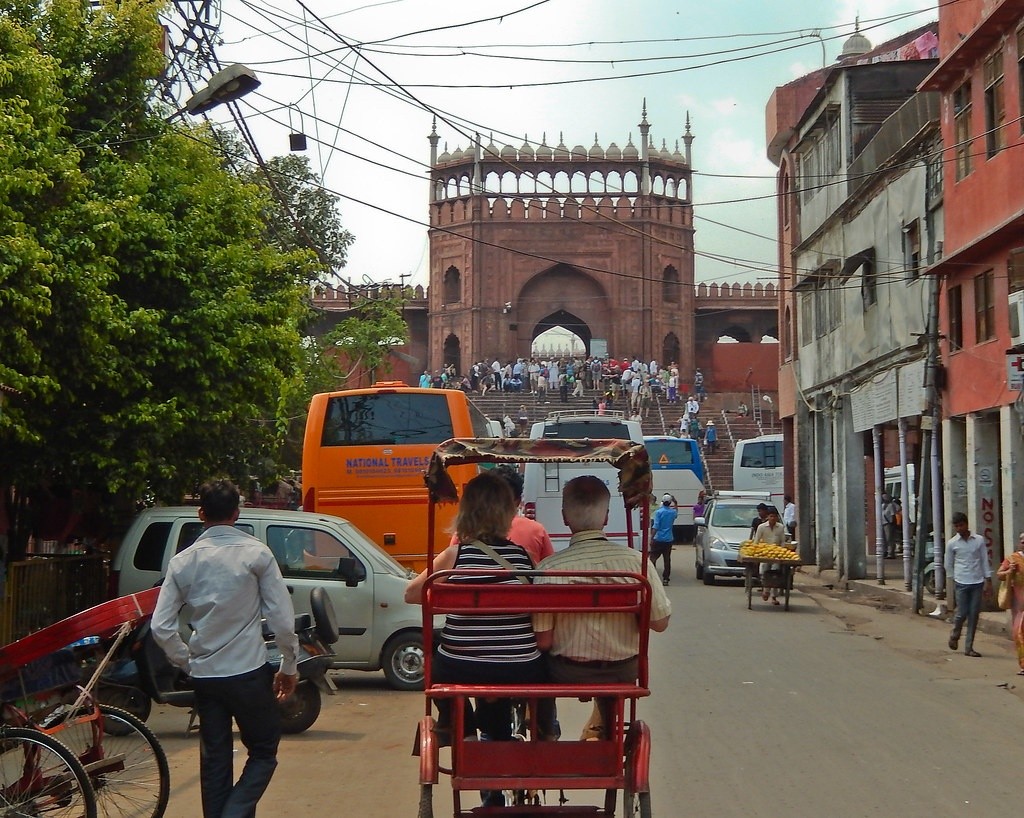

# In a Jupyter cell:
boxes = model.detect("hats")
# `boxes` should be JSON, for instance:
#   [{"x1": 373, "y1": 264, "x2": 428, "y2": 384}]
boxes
[
  {"x1": 624, "y1": 358, "x2": 628, "y2": 361},
  {"x1": 706, "y1": 420, "x2": 715, "y2": 426},
  {"x1": 882, "y1": 494, "x2": 889, "y2": 501},
  {"x1": 662, "y1": 495, "x2": 672, "y2": 503}
]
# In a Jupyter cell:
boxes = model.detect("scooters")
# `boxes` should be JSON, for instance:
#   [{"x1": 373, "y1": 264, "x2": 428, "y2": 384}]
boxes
[{"x1": 84, "y1": 575, "x2": 347, "y2": 736}]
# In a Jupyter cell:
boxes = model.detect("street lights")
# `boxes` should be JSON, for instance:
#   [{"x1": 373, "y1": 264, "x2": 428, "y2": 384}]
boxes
[{"x1": 399, "y1": 273, "x2": 412, "y2": 324}]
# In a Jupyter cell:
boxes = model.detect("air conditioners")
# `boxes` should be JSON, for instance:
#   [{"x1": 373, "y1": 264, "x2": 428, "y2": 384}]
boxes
[{"x1": 1007, "y1": 291, "x2": 1024, "y2": 346}]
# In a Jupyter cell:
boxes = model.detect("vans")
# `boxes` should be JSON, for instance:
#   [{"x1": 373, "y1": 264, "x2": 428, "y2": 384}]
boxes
[{"x1": 106, "y1": 505, "x2": 447, "y2": 692}]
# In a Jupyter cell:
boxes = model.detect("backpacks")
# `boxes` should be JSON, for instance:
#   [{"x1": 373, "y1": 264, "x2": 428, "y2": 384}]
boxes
[
  {"x1": 592, "y1": 360, "x2": 600, "y2": 373},
  {"x1": 697, "y1": 374, "x2": 703, "y2": 384}
]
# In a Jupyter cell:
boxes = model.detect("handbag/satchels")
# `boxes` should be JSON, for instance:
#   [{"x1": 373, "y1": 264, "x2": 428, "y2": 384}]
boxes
[
  {"x1": 895, "y1": 512, "x2": 902, "y2": 527},
  {"x1": 998, "y1": 555, "x2": 1014, "y2": 609},
  {"x1": 788, "y1": 520, "x2": 796, "y2": 527}
]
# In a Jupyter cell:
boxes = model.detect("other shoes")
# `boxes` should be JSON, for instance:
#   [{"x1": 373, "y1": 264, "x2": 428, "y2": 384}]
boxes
[
  {"x1": 762, "y1": 593, "x2": 779, "y2": 605},
  {"x1": 537, "y1": 720, "x2": 560, "y2": 742},
  {"x1": 436, "y1": 718, "x2": 469, "y2": 737},
  {"x1": 949, "y1": 629, "x2": 960, "y2": 650},
  {"x1": 883, "y1": 556, "x2": 895, "y2": 559},
  {"x1": 663, "y1": 580, "x2": 668, "y2": 586},
  {"x1": 965, "y1": 648, "x2": 981, "y2": 657}
]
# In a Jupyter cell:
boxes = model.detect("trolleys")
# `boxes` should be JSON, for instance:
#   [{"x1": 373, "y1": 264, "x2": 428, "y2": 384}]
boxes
[{"x1": 735, "y1": 551, "x2": 806, "y2": 612}]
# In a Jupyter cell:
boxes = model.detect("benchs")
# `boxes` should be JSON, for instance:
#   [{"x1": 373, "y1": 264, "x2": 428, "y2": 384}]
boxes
[{"x1": 421, "y1": 569, "x2": 654, "y2": 697}]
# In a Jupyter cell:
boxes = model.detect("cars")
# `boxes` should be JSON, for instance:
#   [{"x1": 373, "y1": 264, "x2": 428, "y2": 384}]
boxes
[{"x1": 692, "y1": 490, "x2": 793, "y2": 585}]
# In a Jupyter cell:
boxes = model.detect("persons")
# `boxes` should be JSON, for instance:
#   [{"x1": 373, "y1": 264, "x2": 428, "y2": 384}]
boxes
[
  {"x1": 405, "y1": 473, "x2": 542, "y2": 741},
  {"x1": 151, "y1": 476, "x2": 298, "y2": 818},
  {"x1": 586, "y1": 353, "x2": 670, "y2": 426},
  {"x1": 484, "y1": 405, "x2": 527, "y2": 437},
  {"x1": 535, "y1": 476, "x2": 672, "y2": 742},
  {"x1": 420, "y1": 358, "x2": 512, "y2": 396},
  {"x1": 783, "y1": 496, "x2": 794, "y2": 540},
  {"x1": 668, "y1": 361, "x2": 718, "y2": 454},
  {"x1": 650, "y1": 492, "x2": 705, "y2": 586},
  {"x1": 449, "y1": 465, "x2": 554, "y2": 565},
  {"x1": 997, "y1": 531, "x2": 1024, "y2": 675},
  {"x1": 882, "y1": 494, "x2": 902, "y2": 558},
  {"x1": 738, "y1": 401, "x2": 748, "y2": 416},
  {"x1": 944, "y1": 512, "x2": 992, "y2": 657},
  {"x1": 749, "y1": 503, "x2": 785, "y2": 605},
  {"x1": 510, "y1": 356, "x2": 586, "y2": 404}
]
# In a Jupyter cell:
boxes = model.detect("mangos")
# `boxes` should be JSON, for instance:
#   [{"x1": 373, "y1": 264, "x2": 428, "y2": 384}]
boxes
[{"x1": 740, "y1": 539, "x2": 800, "y2": 560}]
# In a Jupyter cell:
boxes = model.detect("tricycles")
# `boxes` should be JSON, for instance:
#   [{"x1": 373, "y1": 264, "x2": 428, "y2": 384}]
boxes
[
  {"x1": 410, "y1": 436, "x2": 658, "y2": 817},
  {"x1": 1, "y1": 587, "x2": 175, "y2": 818}
]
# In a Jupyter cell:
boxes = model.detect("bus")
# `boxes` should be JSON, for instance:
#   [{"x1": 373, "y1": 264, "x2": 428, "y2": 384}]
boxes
[
  {"x1": 732, "y1": 433, "x2": 784, "y2": 516},
  {"x1": 518, "y1": 409, "x2": 653, "y2": 562},
  {"x1": 303, "y1": 381, "x2": 502, "y2": 576},
  {"x1": 641, "y1": 434, "x2": 706, "y2": 543}
]
